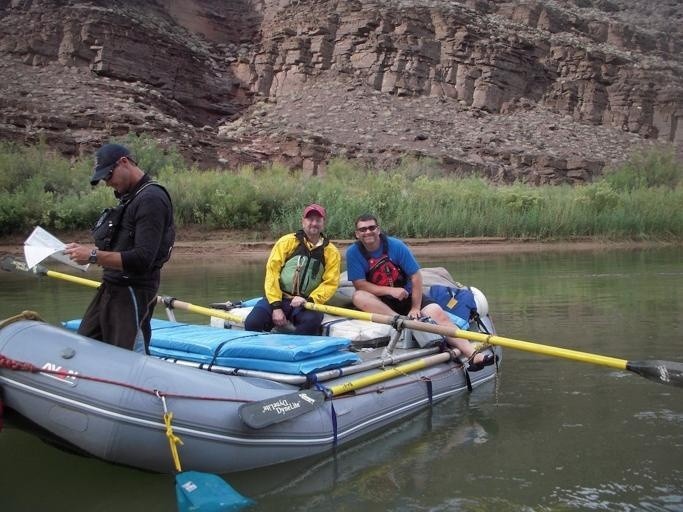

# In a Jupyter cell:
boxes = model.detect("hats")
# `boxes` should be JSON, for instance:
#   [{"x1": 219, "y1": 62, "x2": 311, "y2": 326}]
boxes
[
  {"x1": 304, "y1": 204, "x2": 326, "y2": 218},
  {"x1": 90, "y1": 143, "x2": 130, "y2": 185}
]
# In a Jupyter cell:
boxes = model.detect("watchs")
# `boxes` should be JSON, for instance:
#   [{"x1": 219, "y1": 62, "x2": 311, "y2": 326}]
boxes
[{"x1": 88, "y1": 248, "x2": 97, "y2": 265}]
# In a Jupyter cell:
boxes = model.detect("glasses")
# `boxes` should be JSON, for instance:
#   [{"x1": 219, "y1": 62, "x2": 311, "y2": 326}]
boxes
[
  {"x1": 101, "y1": 170, "x2": 112, "y2": 180},
  {"x1": 358, "y1": 225, "x2": 378, "y2": 232}
]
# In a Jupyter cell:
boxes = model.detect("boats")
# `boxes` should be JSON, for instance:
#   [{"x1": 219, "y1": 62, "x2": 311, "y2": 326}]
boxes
[
  {"x1": 225, "y1": 374, "x2": 504, "y2": 512},
  {"x1": 0, "y1": 265, "x2": 503, "y2": 481}
]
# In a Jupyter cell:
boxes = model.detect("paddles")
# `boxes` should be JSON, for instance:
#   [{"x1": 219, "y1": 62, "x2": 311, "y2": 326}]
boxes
[
  {"x1": 303, "y1": 301, "x2": 683, "y2": 387},
  {"x1": 0, "y1": 255, "x2": 246, "y2": 324}
]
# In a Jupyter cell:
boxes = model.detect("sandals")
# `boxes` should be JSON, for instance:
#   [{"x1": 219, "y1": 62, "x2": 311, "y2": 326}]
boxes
[{"x1": 468, "y1": 351, "x2": 499, "y2": 372}]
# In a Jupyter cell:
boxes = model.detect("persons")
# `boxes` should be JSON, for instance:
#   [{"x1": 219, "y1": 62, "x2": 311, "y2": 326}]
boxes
[
  {"x1": 244, "y1": 204, "x2": 340, "y2": 336},
  {"x1": 346, "y1": 214, "x2": 499, "y2": 372},
  {"x1": 63, "y1": 144, "x2": 174, "y2": 351}
]
